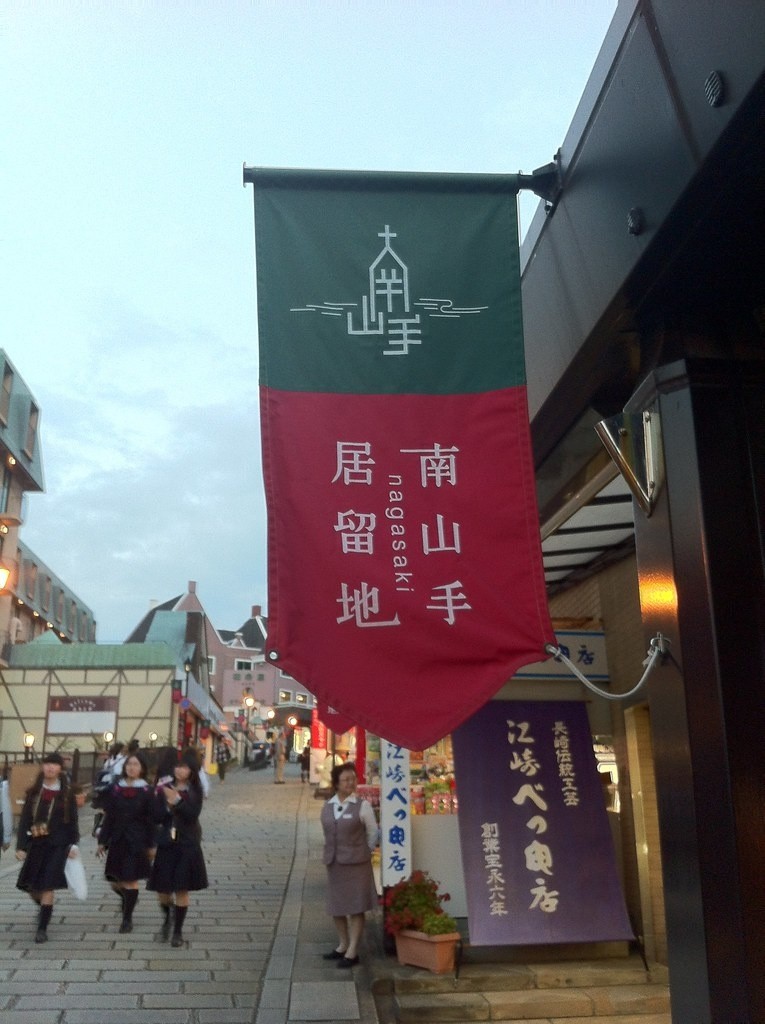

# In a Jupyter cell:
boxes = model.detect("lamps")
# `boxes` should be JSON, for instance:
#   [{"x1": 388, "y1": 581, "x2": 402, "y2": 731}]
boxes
[
  {"x1": 23, "y1": 733, "x2": 35, "y2": 763},
  {"x1": 148, "y1": 732, "x2": 157, "y2": 748}
]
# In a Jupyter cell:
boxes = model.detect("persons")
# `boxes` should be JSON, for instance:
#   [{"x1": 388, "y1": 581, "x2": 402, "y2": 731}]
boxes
[
  {"x1": 155, "y1": 747, "x2": 178, "y2": 773},
  {"x1": 100, "y1": 742, "x2": 128, "y2": 785},
  {"x1": 95, "y1": 754, "x2": 155, "y2": 933},
  {"x1": 182, "y1": 745, "x2": 209, "y2": 800},
  {"x1": 320, "y1": 762, "x2": 379, "y2": 968},
  {"x1": 216, "y1": 735, "x2": 230, "y2": 783},
  {"x1": 128, "y1": 740, "x2": 147, "y2": 778},
  {"x1": 15, "y1": 753, "x2": 80, "y2": 943},
  {"x1": 274, "y1": 733, "x2": 285, "y2": 783},
  {"x1": 145, "y1": 757, "x2": 209, "y2": 947}
]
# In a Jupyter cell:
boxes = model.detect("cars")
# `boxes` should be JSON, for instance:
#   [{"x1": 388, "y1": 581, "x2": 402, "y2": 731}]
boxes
[{"x1": 247, "y1": 740, "x2": 272, "y2": 766}]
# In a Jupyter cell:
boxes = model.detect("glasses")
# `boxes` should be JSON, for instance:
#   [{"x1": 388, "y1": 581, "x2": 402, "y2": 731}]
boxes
[{"x1": 336, "y1": 777, "x2": 356, "y2": 782}]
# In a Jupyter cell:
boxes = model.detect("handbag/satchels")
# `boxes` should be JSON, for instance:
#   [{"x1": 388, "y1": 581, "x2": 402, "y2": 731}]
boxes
[{"x1": 63, "y1": 844, "x2": 89, "y2": 901}]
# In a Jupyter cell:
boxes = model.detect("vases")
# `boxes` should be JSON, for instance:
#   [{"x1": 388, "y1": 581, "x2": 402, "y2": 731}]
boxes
[
  {"x1": 76, "y1": 793, "x2": 85, "y2": 806},
  {"x1": 394, "y1": 928, "x2": 460, "y2": 976}
]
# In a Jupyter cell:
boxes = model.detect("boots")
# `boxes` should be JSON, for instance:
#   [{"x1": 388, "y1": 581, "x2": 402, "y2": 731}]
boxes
[
  {"x1": 159, "y1": 901, "x2": 173, "y2": 939},
  {"x1": 115, "y1": 887, "x2": 139, "y2": 933},
  {"x1": 171, "y1": 905, "x2": 188, "y2": 947},
  {"x1": 36, "y1": 905, "x2": 53, "y2": 942}
]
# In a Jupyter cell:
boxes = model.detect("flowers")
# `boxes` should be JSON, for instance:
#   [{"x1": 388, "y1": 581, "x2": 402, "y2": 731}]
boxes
[
  {"x1": 378, "y1": 870, "x2": 459, "y2": 937},
  {"x1": 71, "y1": 784, "x2": 84, "y2": 795}
]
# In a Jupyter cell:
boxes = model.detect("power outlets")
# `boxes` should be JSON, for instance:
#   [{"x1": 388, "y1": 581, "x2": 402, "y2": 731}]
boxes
[{"x1": 104, "y1": 731, "x2": 114, "y2": 752}]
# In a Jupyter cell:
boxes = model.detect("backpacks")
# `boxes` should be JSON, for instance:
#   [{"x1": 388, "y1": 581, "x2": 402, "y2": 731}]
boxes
[{"x1": 93, "y1": 756, "x2": 123, "y2": 792}]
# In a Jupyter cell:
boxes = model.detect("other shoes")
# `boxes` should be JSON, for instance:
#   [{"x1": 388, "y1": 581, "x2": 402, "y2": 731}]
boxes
[
  {"x1": 337, "y1": 954, "x2": 359, "y2": 968},
  {"x1": 322, "y1": 950, "x2": 346, "y2": 960}
]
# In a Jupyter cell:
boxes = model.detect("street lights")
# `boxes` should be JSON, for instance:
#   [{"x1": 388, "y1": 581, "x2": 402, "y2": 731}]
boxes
[
  {"x1": 242, "y1": 696, "x2": 254, "y2": 769},
  {"x1": 287, "y1": 716, "x2": 298, "y2": 752},
  {"x1": 267, "y1": 709, "x2": 276, "y2": 732}
]
